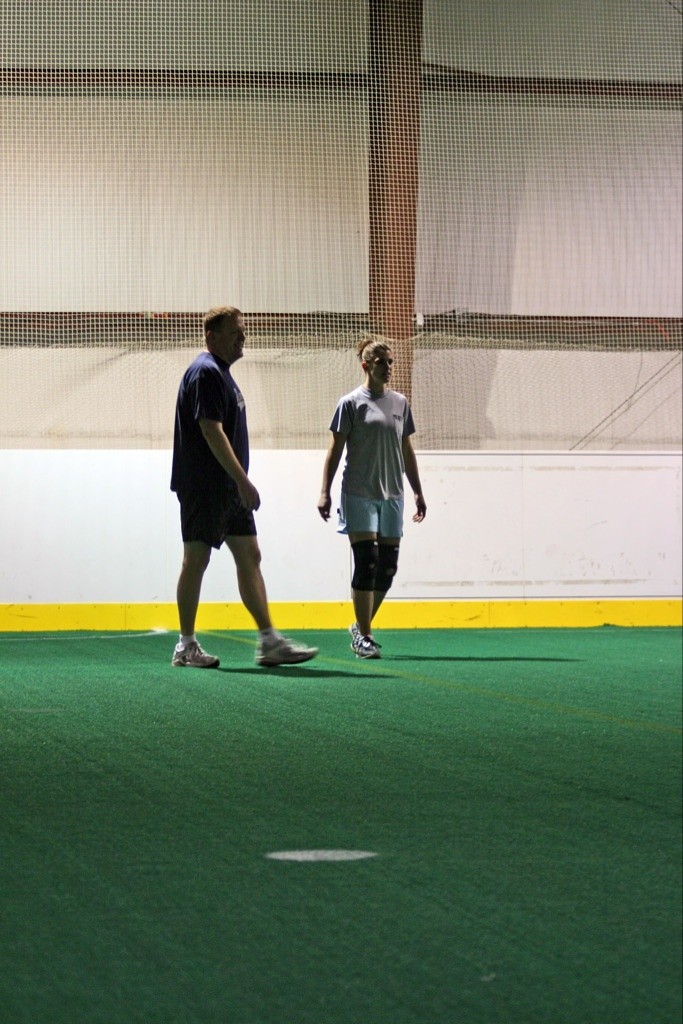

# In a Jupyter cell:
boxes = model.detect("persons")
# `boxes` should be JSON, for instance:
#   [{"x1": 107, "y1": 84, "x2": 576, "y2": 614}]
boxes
[
  {"x1": 171, "y1": 307, "x2": 319, "y2": 668},
  {"x1": 316, "y1": 339, "x2": 427, "y2": 659}
]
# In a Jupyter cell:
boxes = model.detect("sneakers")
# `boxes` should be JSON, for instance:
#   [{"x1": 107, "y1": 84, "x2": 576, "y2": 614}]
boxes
[
  {"x1": 350, "y1": 638, "x2": 383, "y2": 658},
  {"x1": 349, "y1": 624, "x2": 370, "y2": 659},
  {"x1": 171, "y1": 640, "x2": 221, "y2": 668},
  {"x1": 255, "y1": 635, "x2": 319, "y2": 667}
]
